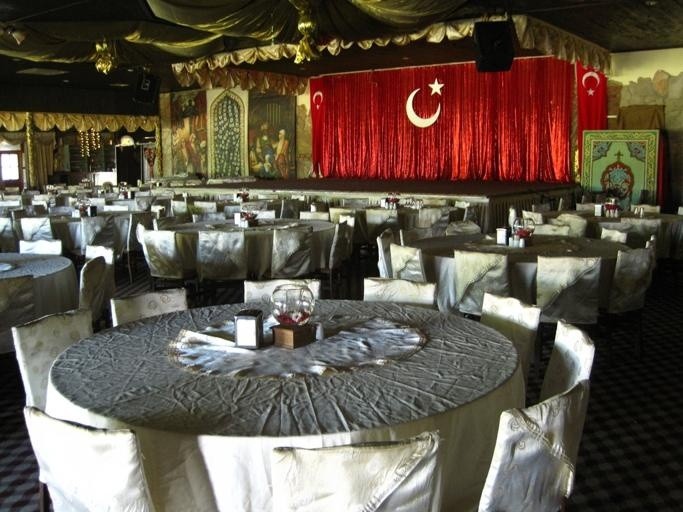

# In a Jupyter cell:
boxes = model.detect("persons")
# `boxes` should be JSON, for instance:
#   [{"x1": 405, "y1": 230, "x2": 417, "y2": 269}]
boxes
[
  {"x1": 175, "y1": 134, "x2": 206, "y2": 178},
  {"x1": 249, "y1": 129, "x2": 290, "y2": 176}
]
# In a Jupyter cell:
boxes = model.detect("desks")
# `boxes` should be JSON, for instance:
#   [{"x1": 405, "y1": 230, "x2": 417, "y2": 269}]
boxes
[{"x1": 46, "y1": 298, "x2": 524, "y2": 511}]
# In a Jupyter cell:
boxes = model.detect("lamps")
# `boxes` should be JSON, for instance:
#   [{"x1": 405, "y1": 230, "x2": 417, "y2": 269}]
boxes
[
  {"x1": 289, "y1": 0, "x2": 319, "y2": 64},
  {"x1": 94, "y1": 28, "x2": 122, "y2": 75}
]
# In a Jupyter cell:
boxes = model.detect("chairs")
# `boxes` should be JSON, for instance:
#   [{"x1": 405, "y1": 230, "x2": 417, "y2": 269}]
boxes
[
  {"x1": 270, "y1": 427, "x2": 444, "y2": 510},
  {"x1": 0, "y1": 179, "x2": 683, "y2": 354},
  {"x1": 21, "y1": 407, "x2": 155, "y2": 511},
  {"x1": 10, "y1": 308, "x2": 95, "y2": 415},
  {"x1": 478, "y1": 381, "x2": 586, "y2": 510},
  {"x1": 543, "y1": 316, "x2": 597, "y2": 406}
]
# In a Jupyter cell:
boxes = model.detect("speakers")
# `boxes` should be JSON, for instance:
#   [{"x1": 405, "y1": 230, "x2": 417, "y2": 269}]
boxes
[
  {"x1": 474, "y1": 21, "x2": 514, "y2": 71},
  {"x1": 133, "y1": 72, "x2": 160, "y2": 104}
]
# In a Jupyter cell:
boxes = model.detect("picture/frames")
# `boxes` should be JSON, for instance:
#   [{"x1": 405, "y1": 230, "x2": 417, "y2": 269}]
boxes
[{"x1": 581, "y1": 129, "x2": 659, "y2": 206}]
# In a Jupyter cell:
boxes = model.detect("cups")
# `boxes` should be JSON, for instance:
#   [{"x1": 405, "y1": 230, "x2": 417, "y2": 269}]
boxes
[{"x1": 252, "y1": 194, "x2": 258, "y2": 201}]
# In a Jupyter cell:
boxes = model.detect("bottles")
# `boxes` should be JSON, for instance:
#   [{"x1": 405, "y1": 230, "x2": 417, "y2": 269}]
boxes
[
  {"x1": 384, "y1": 202, "x2": 396, "y2": 210},
  {"x1": 633, "y1": 207, "x2": 644, "y2": 217},
  {"x1": 605, "y1": 209, "x2": 619, "y2": 220},
  {"x1": 403, "y1": 197, "x2": 423, "y2": 210},
  {"x1": 509, "y1": 235, "x2": 524, "y2": 248},
  {"x1": 315, "y1": 322, "x2": 323, "y2": 339},
  {"x1": 240, "y1": 213, "x2": 253, "y2": 227}
]
